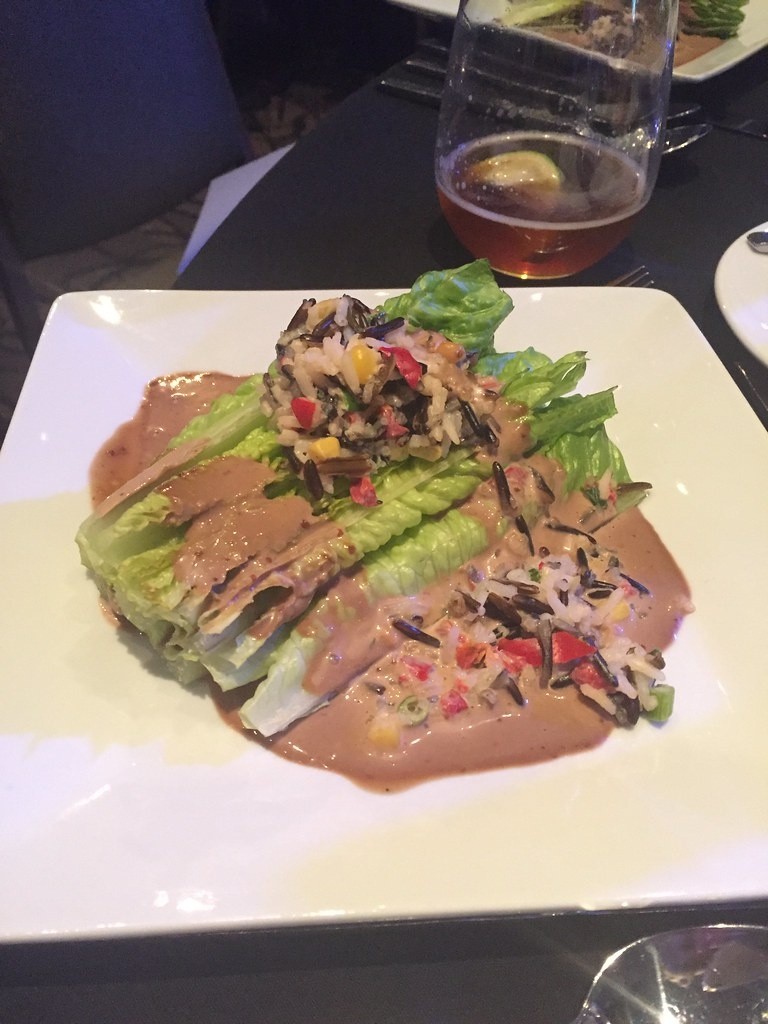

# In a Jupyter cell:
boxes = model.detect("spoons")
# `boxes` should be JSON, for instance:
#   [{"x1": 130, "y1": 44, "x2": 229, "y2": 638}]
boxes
[
  {"x1": 379, "y1": 75, "x2": 711, "y2": 157},
  {"x1": 400, "y1": 55, "x2": 701, "y2": 128}
]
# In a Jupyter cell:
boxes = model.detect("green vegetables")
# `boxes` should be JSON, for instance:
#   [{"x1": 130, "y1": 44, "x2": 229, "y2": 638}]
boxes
[{"x1": 493, "y1": 0, "x2": 749, "y2": 35}]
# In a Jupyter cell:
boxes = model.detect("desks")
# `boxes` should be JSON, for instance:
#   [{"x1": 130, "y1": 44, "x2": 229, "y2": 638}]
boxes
[{"x1": 1, "y1": 26, "x2": 768, "y2": 1022}]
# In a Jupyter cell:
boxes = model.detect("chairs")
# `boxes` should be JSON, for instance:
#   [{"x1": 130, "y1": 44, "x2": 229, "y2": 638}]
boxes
[{"x1": 0, "y1": 0, "x2": 262, "y2": 360}]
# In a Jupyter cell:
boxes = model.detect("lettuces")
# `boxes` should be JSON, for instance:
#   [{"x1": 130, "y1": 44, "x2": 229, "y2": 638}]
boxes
[{"x1": 79, "y1": 257, "x2": 649, "y2": 734}]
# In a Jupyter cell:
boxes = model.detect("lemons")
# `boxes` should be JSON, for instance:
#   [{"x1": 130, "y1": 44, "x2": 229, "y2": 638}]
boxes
[{"x1": 466, "y1": 151, "x2": 561, "y2": 215}]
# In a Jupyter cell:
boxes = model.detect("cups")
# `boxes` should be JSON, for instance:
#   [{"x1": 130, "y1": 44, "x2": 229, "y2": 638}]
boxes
[{"x1": 434, "y1": 0, "x2": 682, "y2": 280}]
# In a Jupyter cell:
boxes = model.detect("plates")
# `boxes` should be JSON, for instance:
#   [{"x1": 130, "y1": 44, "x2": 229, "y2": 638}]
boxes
[
  {"x1": 714, "y1": 221, "x2": 768, "y2": 368},
  {"x1": 0, "y1": 286, "x2": 768, "y2": 945},
  {"x1": 391, "y1": 0, "x2": 768, "y2": 84}
]
